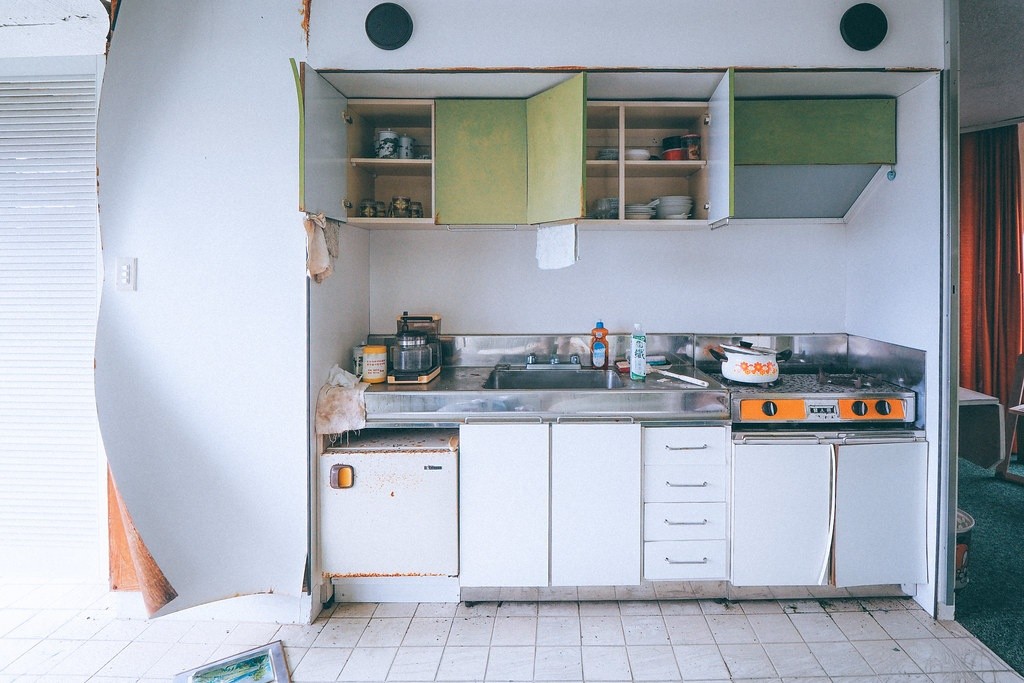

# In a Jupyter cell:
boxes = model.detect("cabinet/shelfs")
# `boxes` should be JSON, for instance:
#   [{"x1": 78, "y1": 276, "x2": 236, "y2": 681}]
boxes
[
  {"x1": 459, "y1": 422, "x2": 928, "y2": 602},
  {"x1": 289, "y1": 58, "x2": 735, "y2": 232},
  {"x1": 319, "y1": 428, "x2": 460, "y2": 603}
]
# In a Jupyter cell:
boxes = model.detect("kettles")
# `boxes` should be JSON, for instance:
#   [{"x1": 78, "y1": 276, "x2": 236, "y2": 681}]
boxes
[{"x1": 388, "y1": 330, "x2": 433, "y2": 373}]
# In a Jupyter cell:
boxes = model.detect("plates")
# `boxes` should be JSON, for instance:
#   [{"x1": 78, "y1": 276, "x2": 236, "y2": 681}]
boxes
[
  {"x1": 625, "y1": 204, "x2": 657, "y2": 219},
  {"x1": 625, "y1": 148, "x2": 650, "y2": 160}
]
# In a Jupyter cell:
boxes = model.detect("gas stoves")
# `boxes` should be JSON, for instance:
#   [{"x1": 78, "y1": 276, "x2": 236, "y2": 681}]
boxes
[{"x1": 709, "y1": 372, "x2": 918, "y2": 431}]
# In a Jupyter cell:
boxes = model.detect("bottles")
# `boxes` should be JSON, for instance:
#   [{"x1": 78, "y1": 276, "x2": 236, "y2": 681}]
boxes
[
  {"x1": 589, "y1": 321, "x2": 609, "y2": 370},
  {"x1": 630, "y1": 324, "x2": 648, "y2": 383}
]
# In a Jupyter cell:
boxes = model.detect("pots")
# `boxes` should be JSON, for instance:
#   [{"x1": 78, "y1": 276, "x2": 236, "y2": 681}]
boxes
[{"x1": 709, "y1": 340, "x2": 792, "y2": 384}]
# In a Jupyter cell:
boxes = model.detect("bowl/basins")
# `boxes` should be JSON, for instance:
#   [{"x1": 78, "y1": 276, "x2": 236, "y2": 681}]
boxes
[
  {"x1": 598, "y1": 149, "x2": 619, "y2": 160},
  {"x1": 660, "y1": 148, "x2": 688, "y2": 160},
  {"x1": 605, "y1": 198, "x2": 618, "y2": 218},
  {"x1": 652, "y1": 196, "x2": 694, "y2": 220}
]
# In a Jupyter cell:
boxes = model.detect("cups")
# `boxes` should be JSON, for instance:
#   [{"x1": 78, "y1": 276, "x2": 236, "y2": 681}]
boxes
[
  {"x1": 352, "y1": 341, "x2": 368, "y2": 382},
  {"x1": 680, "y1": 134, "x2": 700, "y2": 159},
  {"x1": 372, "y1": 135, "x2": 379, "y2": 157},
  {"x1": 378, "y1": 128, "x2": 399, "y2": 158},
  {"x1": 595, "y1": 198, "x2": 611, "y2": 219},
  {"x1": 392, "y1": 196, "x2": 410, "y2": 217},
  {"x1": 360, "y1": 199, "x2": 377, "y2": 217},
  {"x1": 399, "y1": 133, "x2": 415, "y2": 159},
  {"x1": 375, "y1": 201, "x2": 386, "y2": 217},
  {"x1": 410, "y1": 201, "x2": 423, "y2": 218}
]
